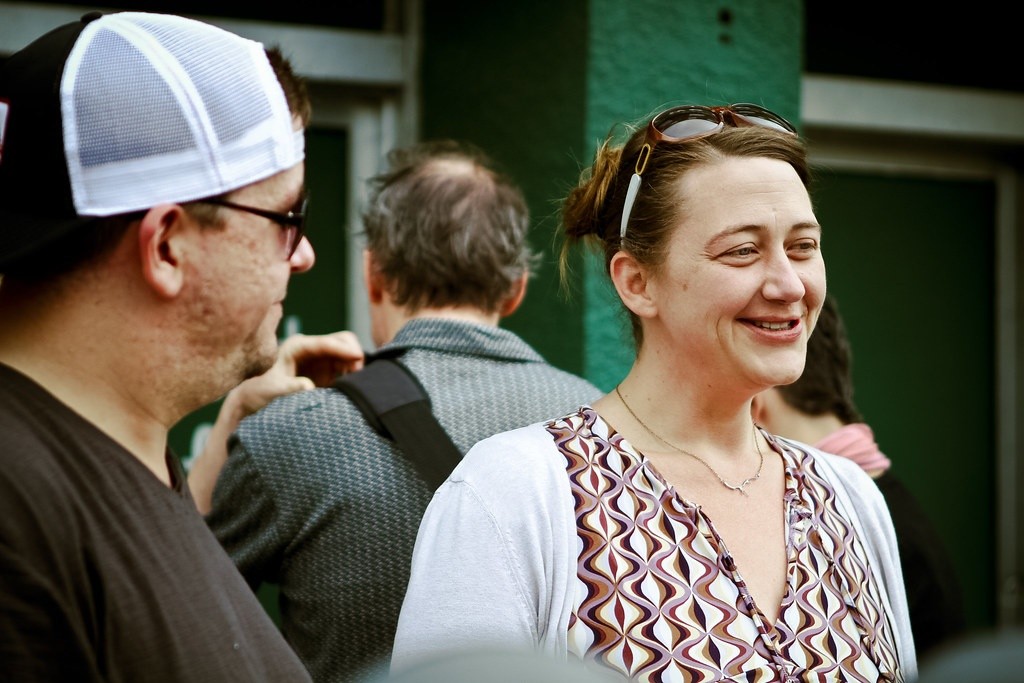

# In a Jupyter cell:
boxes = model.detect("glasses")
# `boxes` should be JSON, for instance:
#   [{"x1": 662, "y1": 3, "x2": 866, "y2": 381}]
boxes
[
  {"x1": 196, "y1": 197, "x2": 310, "y2": 261},
  {"x1": 618, "y1": 103, "x2": 798, "y2": 250}
]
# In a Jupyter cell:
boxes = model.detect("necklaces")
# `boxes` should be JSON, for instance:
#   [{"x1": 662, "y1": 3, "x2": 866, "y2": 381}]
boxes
[{"x1": 615, "y1": 384, "x2": 763, "y2": 498}]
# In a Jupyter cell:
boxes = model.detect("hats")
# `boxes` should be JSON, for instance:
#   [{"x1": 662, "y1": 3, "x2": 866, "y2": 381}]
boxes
[{"x1": 0, "y1": 9, "x2": 305, "y2": 286}]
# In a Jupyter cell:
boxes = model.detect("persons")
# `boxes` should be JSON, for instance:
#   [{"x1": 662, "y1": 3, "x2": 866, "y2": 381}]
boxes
[
  {"x1": 185, "y1": 138, "x2": 608, "y2": 683},
  {"x1": 752, "y1": 294, "x2": 947, "y2": 666},
  {"x1": 0, "y1": 10, "x2": 317, "y2": 683},
  {"x1": 389, "y1": 103, "x2": 920, "y2": 682}
]
